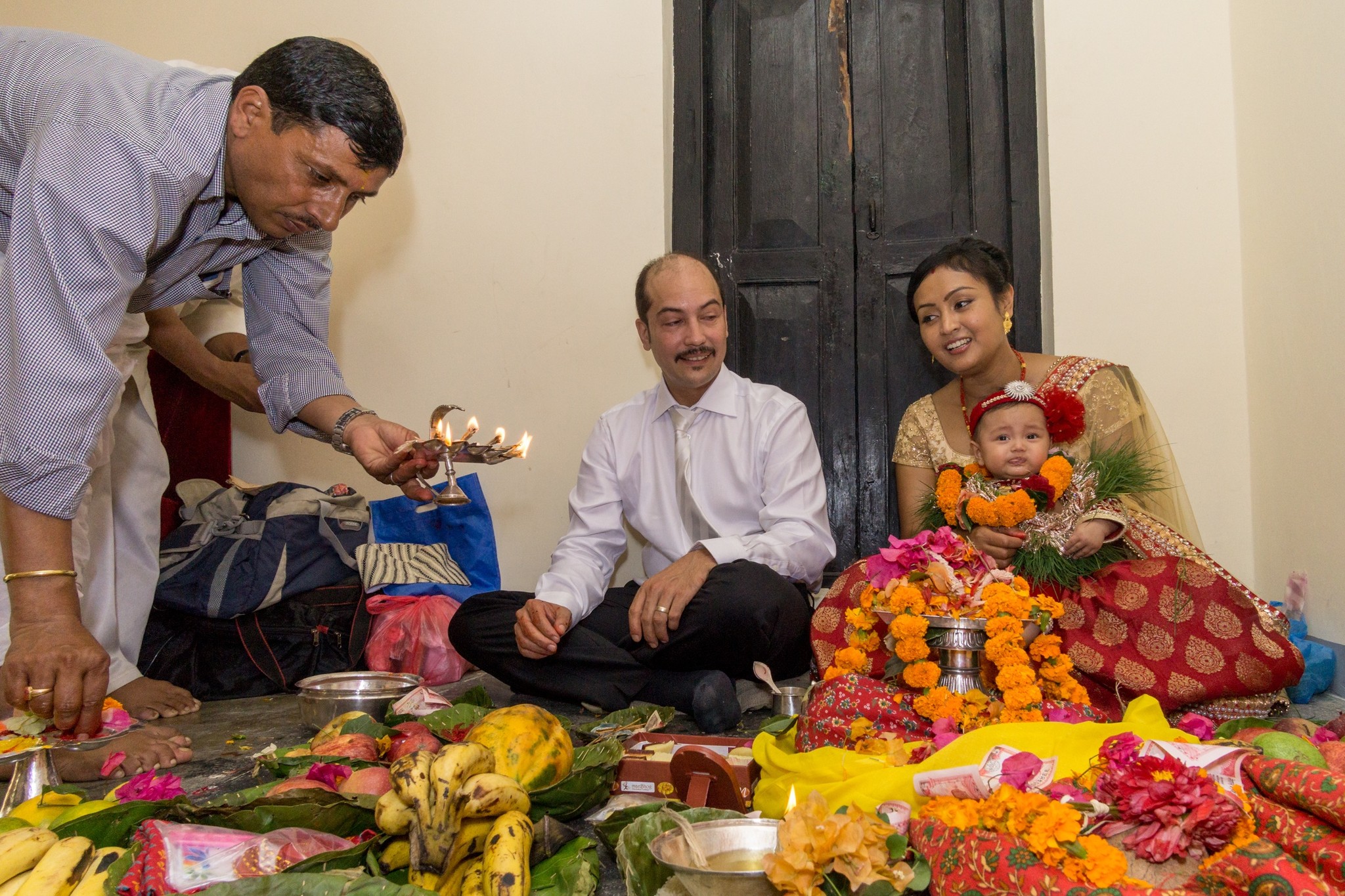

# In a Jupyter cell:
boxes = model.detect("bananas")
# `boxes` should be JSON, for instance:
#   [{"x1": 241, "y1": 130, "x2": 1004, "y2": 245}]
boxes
[
  {"x1": 0, "y1": 793, "x2": 123, "y2": 896},
  {"x1": 360, "y1": 740, "x2": 534, "y2": 896}
]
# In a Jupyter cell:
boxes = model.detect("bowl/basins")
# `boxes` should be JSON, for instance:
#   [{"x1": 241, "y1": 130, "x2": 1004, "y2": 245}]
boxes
[
  {"x1": 295, "y1": 671, "x2": 425, "y2": 731},
  {"x1": 770, "y1": 686, "x2": 808, "y2": 716},
  {"x1": 651, "y1": 818, "x2": 787, "y2": 896}
]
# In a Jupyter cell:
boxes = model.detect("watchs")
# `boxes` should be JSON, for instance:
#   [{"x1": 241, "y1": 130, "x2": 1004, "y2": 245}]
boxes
[{"x1": 330, "y1": 405, "x2": 379, "y2": 456}]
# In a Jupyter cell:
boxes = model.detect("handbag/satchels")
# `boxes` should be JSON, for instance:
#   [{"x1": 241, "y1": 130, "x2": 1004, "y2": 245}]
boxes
[{"x1": 135, "y1": 472, "x2": 501, "y2": 701}]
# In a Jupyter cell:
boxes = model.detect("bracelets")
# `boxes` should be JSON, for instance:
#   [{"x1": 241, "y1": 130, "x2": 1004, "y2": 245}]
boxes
[
  {"x1": 1, "y1": 566, "x2": 82, "y2": 583},
  {"x1": 228, "y1": 348, "x2": 249, "y2": 363}
]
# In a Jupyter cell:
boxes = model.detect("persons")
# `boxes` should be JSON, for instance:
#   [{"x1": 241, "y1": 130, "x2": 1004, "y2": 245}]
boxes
[
  {"x1": 0, "y1": 17, "x2": 443, "y2": 788},
  {"x1": 800, "y1": 236, "x2": 1306, "y2": 755},
  {"x1": 144, "y1": 279, "x2": 272, "y2": 552},
  {"x1": 445, "y1": 251, "x2": 840, "y2": 736},
  {"x1": 943, "y1": 381, "x2": 1132, "y2": 573}
]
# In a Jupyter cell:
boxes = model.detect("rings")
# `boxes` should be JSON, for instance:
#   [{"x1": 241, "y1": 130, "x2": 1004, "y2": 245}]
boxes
[
  {"x1": 656, "y1": 605, "x2": 670, "y2": 614},
  {"x1": 22, "y1": 686, "x2": 55, "y2": 701},
  {"x1": 390, "y1": 471, "x2": 405, "y2": 486}
]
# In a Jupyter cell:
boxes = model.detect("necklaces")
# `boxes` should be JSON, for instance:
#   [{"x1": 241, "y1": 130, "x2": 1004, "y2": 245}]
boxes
[{"x1": 958, "y1": 340, "x2": 1026, "y2": 441}]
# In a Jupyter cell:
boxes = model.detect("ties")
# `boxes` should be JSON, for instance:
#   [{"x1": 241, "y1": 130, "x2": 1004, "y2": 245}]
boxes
[{"x1": 668, "y1": 407, "x2": 724, "y2": 543}]
